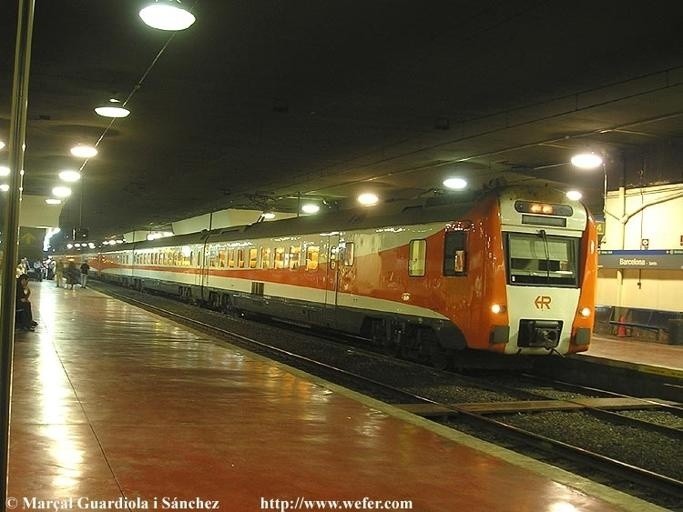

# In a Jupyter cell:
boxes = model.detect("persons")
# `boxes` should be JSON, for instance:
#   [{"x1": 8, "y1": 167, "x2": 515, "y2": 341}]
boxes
[
  {"x1": 14, "y1": 254, "x2": 54, "y2": 283},
  {"x1": 15, "y1": 274, "x2": 37, "y2": 331},
  {"x1": 78, "y1": 260, "x2": 89, "y2": 289},
  {"x1": 65, "y1": 258, "x2": 75, "y2": 290},
  {"x1": 53, "y1": 258, "x2": 63, "y2": 288}
]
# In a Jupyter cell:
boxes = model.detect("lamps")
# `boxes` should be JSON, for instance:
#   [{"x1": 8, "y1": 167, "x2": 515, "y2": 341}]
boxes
[{"x1": 91, "y1": 95, "x2": 132, "y2": 119}]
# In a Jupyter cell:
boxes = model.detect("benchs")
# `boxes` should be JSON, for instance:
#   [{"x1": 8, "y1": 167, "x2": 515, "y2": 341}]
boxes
[{"x1": 608, "y1": 321, "x2": 670, "y2": 343}]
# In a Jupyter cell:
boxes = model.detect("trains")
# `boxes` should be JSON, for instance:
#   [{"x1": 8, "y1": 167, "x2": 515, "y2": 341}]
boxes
[{"x1": 47, "y1": 183, "x2": 598, "y2": 371}]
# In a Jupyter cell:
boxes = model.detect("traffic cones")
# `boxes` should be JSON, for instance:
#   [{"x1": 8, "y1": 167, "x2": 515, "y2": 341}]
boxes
[{"x1": 616, "y1": 315, "x2": 626, "y2": 336}]
